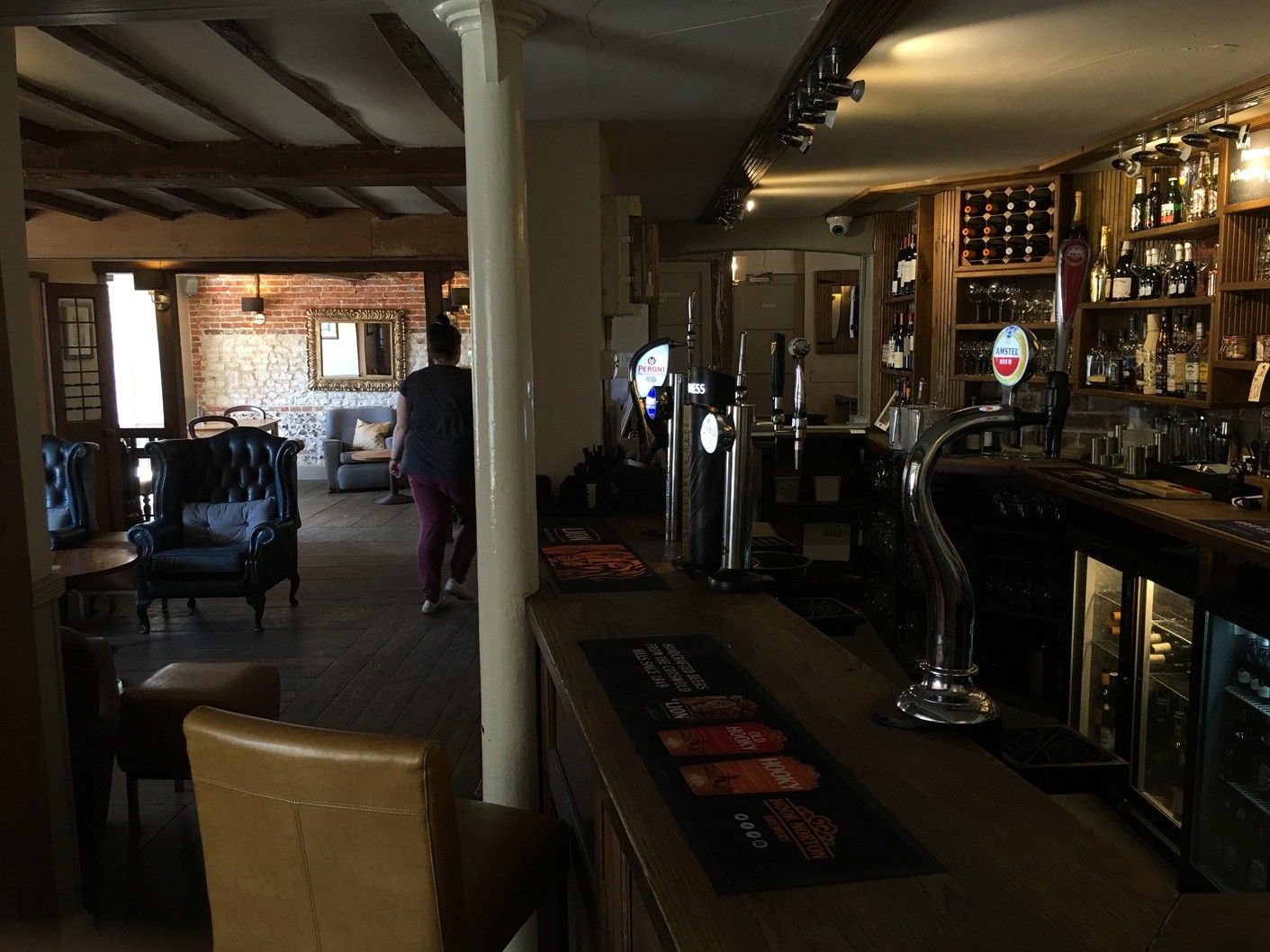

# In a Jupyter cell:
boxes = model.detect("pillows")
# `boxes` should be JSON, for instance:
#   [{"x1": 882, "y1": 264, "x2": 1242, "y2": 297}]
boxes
[{"x1": 353, "y1": 417, "x2": 392, "y2": 450}]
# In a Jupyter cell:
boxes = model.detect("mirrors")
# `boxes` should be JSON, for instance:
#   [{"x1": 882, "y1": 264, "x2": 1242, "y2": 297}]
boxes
[{"x1": 304, "y1": 308, "x2": 406, "y2": 391}]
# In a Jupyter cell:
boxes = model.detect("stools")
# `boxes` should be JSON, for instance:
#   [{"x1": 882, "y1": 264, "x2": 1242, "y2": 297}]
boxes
[{"x1": 113, "y1": 660, "x2": 280, "y2": 832}]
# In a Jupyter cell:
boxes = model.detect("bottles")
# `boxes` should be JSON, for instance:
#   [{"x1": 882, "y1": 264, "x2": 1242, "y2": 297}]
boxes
[
  {"x1": 982, "y1": 245, "x2": 1006, "y2": 259},
  {"x1": 983, "y1": 226, "x2": 1006, "y2": 237},
  {"x1": 966, "y1": 395, "x2": 1002, "y2": 458},
  {"x1": 1092, "y1": 668, "x2": 1188, "y2": 823},
  {"x1": 1067, "y1": 190, "x2": 1089, "y2": 243},
  {"x1": 1237, "y1": 630, "x2": 1270, "y2": 705},
  {"x1": 1085, "y1": 308, "x2": 1209, "y2": 401},
  {"x1": 962, "y1": 249, "x2": 985, "y2": 260},
  {"x1": 1090, "y1": 223, "x2": 1219, "y2": 302},
  {"x1": 1026, "y1": 222, "x2": 1050, "y2": 234},
  {"x1": 985, "y1": 203, "x2": 1007, "y2": 214},
  {"x1": 888, "y1": 313, "x2": 914, "y2": 371},
  {"x1": 1006, "y1": 201, "x2": 1030, "y2": 212},
  {"x1": 895, "y1": 377, "x2": 912, "y2": 405},
  {"x1": 1108, "y1": 612, "x2": 1192, "y2": 678},
  {"x1": 1208, "y1": 695, "x2": 1270, "y2": 893},
  {"x1": 1130, "y1": 150, "x2": 1219, "y2": 233},
  {"x1": 1028, "y1": 199, "x2": 1051, "y2": 210},
  {"x1": 964, "y1": 206, "x2": 986, "y2": 216},
  {"x1": 1005, "y1": 223, "x2": 1028, "y2": 236},
  {"x1": 1025, "y1": 243, "x2": 1050, "y2": 256},
  {"x1": 1005, "y1": 244, "x2": 1027, "y2": 258},
  {"x1": 962, "y1": 228, "x2": 984, "y2": 238},
  {"x1": 892, "y1": 233, "x2": 916, "y2": 298}
]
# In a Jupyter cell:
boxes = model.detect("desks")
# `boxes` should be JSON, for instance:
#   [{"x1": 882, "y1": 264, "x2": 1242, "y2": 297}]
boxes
[
  {"x1": 351, "y1": 451, "x2": 415, "y2": 504},
  {"x1": 48, "y1": 531, "x2": 140, "y2": 651},
  {"x1": 190, "y1": 418, "x2": 283, "y2": 436}
]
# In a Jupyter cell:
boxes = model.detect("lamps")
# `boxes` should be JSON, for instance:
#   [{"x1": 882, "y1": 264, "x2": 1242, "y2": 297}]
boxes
[
  {"x1": 1131, "y1": 133, "x2": 1160, "y2": 163},
  {"x1": 242, "y1": 297, "x2": 266, "y2": 325},
  {"x1": 717, "y1": 189, "x2": 754, "y2": 233},
  {"x1": 1154, "y1": 123, "x2": 1193, "y2": 162},
  {"x1": 1111, "y1": 141, "x2": 1140, "y2": 177},
  {"x1": 443, "y1": 288, "x2": 470, "y2": 325},
  {"x1": 1210, "y1": 100, "x2": 1252, "y2": 142},
  {"x1": 1181, "y1": 113, "x2": 1211, "y2": 148},
  {"x1": 781, "y1": 44, "x2": 866, "y2": 155}
]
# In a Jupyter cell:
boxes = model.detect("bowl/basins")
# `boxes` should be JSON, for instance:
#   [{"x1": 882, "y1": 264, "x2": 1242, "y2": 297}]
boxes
[{"x1": 751, "y1": 551, "x2": 812, "y2": 588}]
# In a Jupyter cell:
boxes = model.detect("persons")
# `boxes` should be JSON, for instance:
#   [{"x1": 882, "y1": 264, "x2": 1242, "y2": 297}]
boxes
[{"x1": 387, "y1": 324, "x2": 475, "y2": 612}]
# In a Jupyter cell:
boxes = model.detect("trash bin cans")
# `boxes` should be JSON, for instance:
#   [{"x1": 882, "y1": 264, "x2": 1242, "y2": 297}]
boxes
[{"x1": 750, "y1": 551, "x2": 812, "y2": 584}]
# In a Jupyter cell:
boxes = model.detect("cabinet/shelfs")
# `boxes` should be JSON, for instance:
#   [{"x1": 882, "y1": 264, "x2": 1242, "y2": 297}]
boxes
[{"x1": 883, "y1": 82, "x2": 1270, "y2": 909}]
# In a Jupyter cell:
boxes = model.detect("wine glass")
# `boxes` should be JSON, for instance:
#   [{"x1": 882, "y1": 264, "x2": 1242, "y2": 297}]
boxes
[
  {"x1": 969, "y1": 280, "x2": 1053, "y2": 323},
  {"x1": 979, "y1": 549, "x2": 1064, "y2": 609},
  {"x1": 959, "y1": 341, "x2": 1072, "y2": 377}
]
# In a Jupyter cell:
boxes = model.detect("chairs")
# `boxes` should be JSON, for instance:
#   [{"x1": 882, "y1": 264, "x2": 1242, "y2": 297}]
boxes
[
  {"x1": 125, "y1": 428, "x2": 307, "y2": 636},
  {"x1": 185, "y1": 709, "x2": 565, "y2": 952},
  {"x1": 40, "y1": 434, "x2": 100, "y2": 550}
]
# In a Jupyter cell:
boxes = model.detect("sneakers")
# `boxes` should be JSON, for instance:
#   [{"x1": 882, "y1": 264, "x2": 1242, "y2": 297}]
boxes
[
  {"x1": 444, "y1": 578, "x2": 475, "y2": 601},
  {"x1": 422, "y1": 596, "x2": 449, "y2": 614}
]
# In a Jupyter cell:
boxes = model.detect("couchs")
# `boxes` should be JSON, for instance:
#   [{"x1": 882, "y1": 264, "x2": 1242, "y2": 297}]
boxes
[{"x1": 323, "y1": 407, "x2": 401, "y2": 493}]
